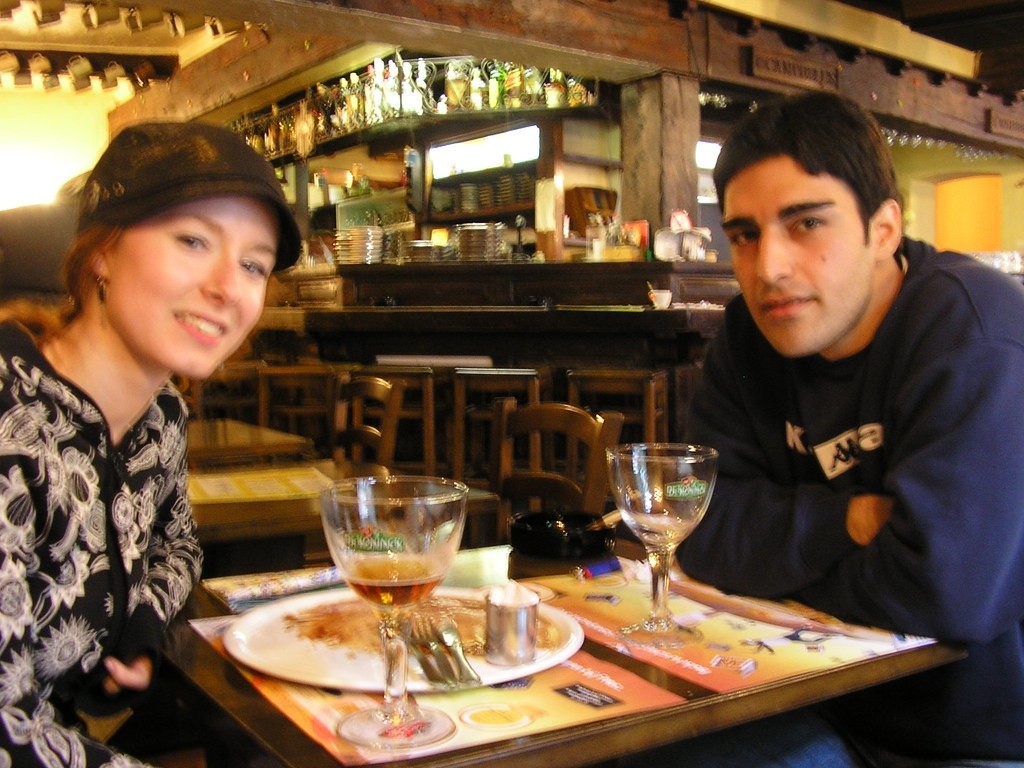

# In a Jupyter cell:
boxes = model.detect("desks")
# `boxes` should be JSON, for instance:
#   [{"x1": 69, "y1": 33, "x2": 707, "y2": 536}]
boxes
[
  {"x1": 161, "y1": 559, "x2": 967, "y2": 767},
  {"x1": 189, "y1": 453, "x2": 502, "y2": 542},
  {"x1": 187, "y1": 414, "x2": 315, "y2": 467}
]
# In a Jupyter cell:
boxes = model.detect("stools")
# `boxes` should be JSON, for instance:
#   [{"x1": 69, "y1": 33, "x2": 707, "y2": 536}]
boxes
[{"x1": 189, "y1": 360, "x2": 668, "y2": 518}]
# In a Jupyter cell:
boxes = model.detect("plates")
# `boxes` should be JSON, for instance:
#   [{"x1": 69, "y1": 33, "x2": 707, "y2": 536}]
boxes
[
  {"x1": 222, "y1": 588, "x2": 583, "y2": 692},
  {"x1": 333, "y1": 224, "x2": 381, "y2": 264},
  {"x1": 381, "y1": 222, "x2": 511, "y2": 265}
]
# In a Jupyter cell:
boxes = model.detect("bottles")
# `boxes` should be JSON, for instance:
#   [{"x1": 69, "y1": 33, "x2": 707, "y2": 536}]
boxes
[
  {"x1": 222, "y1": 48, "x2": 595, "y2": 157},
  {"x1": 585, "y1": 209, "x2": 711, "y2": 263}
]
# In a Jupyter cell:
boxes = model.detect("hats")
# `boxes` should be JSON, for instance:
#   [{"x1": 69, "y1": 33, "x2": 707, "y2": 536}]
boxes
[{"x1": 79, "y1": 122, "x2": 302, "y2": 273}]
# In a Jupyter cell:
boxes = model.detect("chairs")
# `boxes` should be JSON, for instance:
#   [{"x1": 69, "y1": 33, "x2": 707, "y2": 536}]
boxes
[
  {"x1": 492, "y1": 397, "x2": 625, "y2": 556},
  {"x1": 307, "y1": 371, "x2": 409, "y2": 563}
]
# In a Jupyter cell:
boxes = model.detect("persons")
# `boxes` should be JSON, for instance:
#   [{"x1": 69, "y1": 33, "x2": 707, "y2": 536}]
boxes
[
  {"x1": 0, "y1": 122, "x2": 305, "y2": 768},
  {"x1": 674, "y1": 91, "x2": 1024, "y2": 768}
]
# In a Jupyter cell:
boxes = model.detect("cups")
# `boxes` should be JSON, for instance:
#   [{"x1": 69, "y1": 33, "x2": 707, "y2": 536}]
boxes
[
  {"x1": 485, "y1": 596, "x2": 538, "y2": 665},
  {"x1": 648, "y1": 289, "x2": 672, "y2": 309}
]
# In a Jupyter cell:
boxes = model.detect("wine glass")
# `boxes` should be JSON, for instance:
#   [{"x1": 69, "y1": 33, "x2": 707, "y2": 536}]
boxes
[
  {"x1": 604, "y1": 442, "x2": 719, "y2": 649},
  {"x1": 319, "y1": 474, "x2": 467, "y2": 748}
]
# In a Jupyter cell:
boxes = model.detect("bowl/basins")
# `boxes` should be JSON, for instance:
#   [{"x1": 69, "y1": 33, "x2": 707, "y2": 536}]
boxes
[{"x1": 460, "y1": 172, "x2": 534, "y2": 213}]
